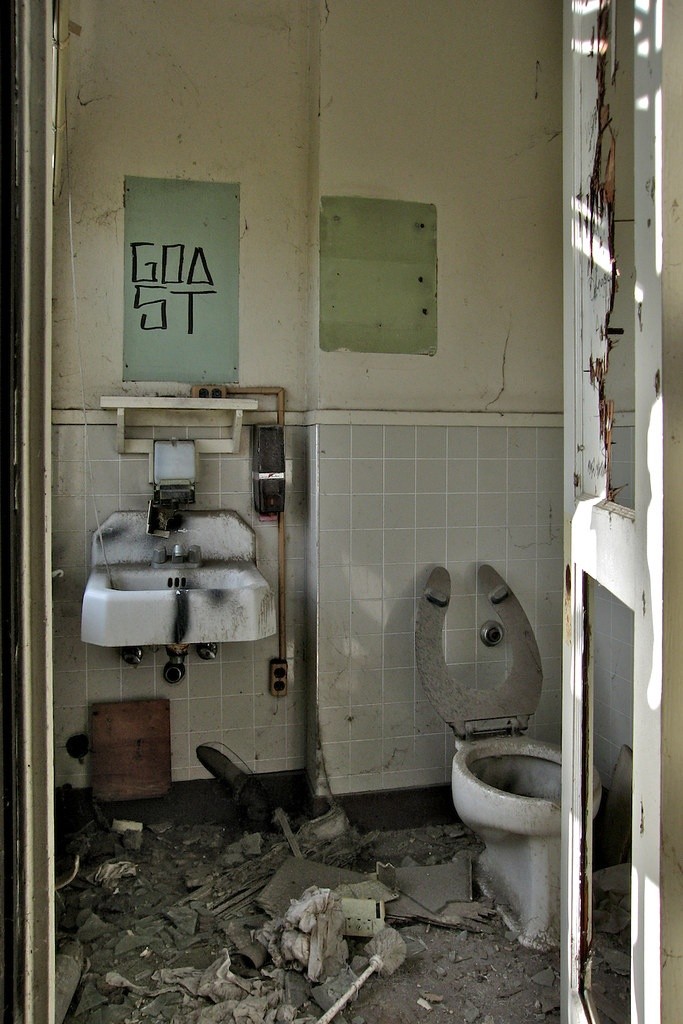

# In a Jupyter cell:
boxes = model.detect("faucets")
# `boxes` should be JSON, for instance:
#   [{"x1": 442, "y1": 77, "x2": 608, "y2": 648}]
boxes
[{"x1": 151, "y1": 543, "x2": 203, "y2": 570}]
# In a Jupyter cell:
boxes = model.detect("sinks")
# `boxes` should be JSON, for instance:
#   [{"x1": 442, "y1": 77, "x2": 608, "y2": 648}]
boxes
[{"x1": 80, "y1": 509, "x2": 278, "y2": 648}]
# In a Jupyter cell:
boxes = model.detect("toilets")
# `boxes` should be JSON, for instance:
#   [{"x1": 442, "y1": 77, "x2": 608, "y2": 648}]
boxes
[{"x1": 414, "y1": 563, "x2": 604, "y2": 955}]
